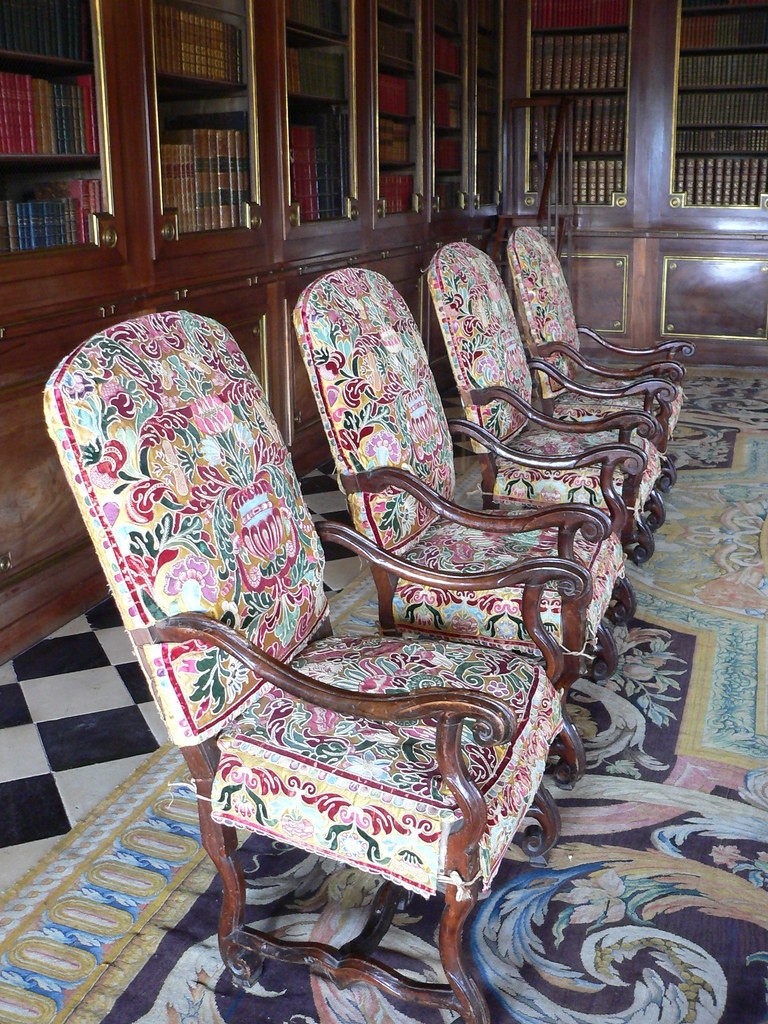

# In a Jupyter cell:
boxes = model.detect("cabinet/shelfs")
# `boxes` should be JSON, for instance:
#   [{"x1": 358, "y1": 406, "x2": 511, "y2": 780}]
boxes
[
  {"x1": 502, "y1": 0, "x2": 768, "y2": 366},
  {"x1": 0, "y1": 0, "x2": 512, "y2": 669}
]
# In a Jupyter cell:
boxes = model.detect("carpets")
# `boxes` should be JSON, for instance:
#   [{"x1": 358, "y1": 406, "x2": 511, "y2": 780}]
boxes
[{"x1": 1, "y1": 359, "x2": 768, "y2": 1024}]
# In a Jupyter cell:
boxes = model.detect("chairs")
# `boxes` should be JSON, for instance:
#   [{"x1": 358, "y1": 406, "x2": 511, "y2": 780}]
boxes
[
  {"x1": 291, "y1": 266, "x2": 638, "y2": 741},
  {"x1": 42, "y1": 312, "x2": 592, "y2": 1024},
  {"x1": 510, "y1": 225, "x2": 693, "y2": 494},
  {"x1": 431, "y1": 240, "x2": 670, "y2": 562}
]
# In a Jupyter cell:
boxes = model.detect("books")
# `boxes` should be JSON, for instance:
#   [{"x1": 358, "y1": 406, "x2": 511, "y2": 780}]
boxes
[
  {"x1": 681, "y1": 0, "x2": 768, "y2": 48},
  {"x1": 378, "y1": 0, "x2": 415, "y2": 213},
  {"x1": 290, "y1": 105, "x2": 350, "y2": 221},
  {"x1": 153, "y1": 2, "x2": 243, "y2": 82},
  {"x1": 160, "y1": 111, "x2": 251, "y2": 232},
  {"x1": 287, "y1": 47, "x2": 344, "y2": 98},
  {"x1": 0, "y1": 0, "x2": 94, "y2": 62},
  {"x1": 531, "y1": 0, "x2": 628, "y2": 28},
  {"x1": 676, "y1": 156, "x2": 768, "y2": 206},
  {"x1": 478, "y1": 0, "x2": 497, "y2": 203},
  {"x1": 679, "y1": 54, "x2": 768, "y2": 86},
  {"x1": 0, "y1": 179, "x2": 103, "y2": 252},
  {"x1": 676, "y1": 129, "x2": 768, "y2": 151},
  {"x1": 678, "y1": 93, "x2": 768, "y2": 124},
  {"x1": 531, "y1": 95, "x2": 626, "y2": 151},
  {"x1": 529, "y1": 159, "x2": 623, "y2": 204},
  {"x1": 435, "y1": 0, "x2": 462, "y2": 208},
  {"x1": 286, "y1": 0, "x2": 342, "y2": 33},
  {"x1": 0, "y1": 72, "x2": 98, "y2": 154},
  {"x1": 530, "y1": 33, "x2": 628, "y2": 90}
]
